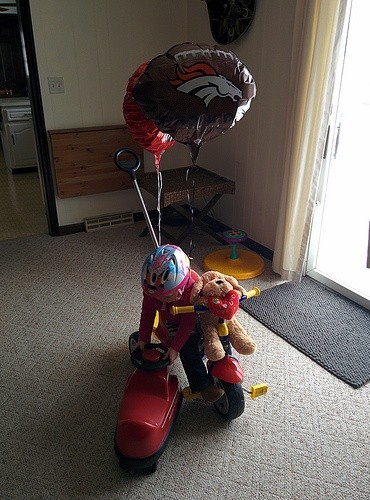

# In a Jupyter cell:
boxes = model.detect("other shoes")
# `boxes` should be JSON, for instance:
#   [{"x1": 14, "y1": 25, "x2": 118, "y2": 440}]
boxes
[{"x1": 202, "y1": 385, "x2": 224, "y2": 404}]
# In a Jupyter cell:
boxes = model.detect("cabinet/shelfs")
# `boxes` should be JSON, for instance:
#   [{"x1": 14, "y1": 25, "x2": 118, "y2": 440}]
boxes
[{"x1": 0, "y1": 97, "x2": 39, "y2": 174}]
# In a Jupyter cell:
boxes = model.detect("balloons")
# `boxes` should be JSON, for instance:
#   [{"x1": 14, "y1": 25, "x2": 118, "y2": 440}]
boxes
[
  {"x1": 121, "y1": 62, "x2": 177, "y2": 153},
  {"x1": 131, "y1": 42, "x2": 257, "y2": 160}
]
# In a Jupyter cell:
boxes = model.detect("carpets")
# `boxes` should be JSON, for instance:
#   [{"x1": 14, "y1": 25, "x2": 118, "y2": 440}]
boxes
[{"x1": 238, "y1": 276, "x2": 370, "y2": 388}]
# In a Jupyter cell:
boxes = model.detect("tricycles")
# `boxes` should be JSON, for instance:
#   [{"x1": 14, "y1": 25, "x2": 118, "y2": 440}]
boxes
[{"x1": 128, "y1": 280, "x2": 261, "y2": 419}]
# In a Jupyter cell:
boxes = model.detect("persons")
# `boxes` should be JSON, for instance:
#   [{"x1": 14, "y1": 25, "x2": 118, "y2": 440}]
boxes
[{"x1": 131, "y1": 243, "x2": 226, "y2": 403}]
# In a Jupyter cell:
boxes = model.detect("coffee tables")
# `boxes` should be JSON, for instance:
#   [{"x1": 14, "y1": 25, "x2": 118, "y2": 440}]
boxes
[{"x1": 133, "y1": 165, "x2": 237, "y2": 250}]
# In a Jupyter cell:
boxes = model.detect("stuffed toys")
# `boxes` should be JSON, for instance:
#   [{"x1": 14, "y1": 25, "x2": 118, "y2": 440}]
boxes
[{"x1": 190, "y1": 270, "x2": 256, "y2": 361}]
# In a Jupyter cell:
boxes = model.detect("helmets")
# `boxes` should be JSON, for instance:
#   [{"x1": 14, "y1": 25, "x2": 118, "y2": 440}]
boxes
[{"x1": 140, "y1": 243, "x2": 190, "y2": 298}]
